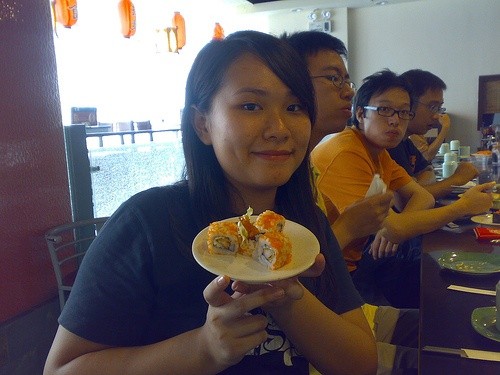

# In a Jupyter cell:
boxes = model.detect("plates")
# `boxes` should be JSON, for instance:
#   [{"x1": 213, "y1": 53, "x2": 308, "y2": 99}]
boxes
[
  {"x1": 191, "y1": 214, "x2": 320, "y2": 283},
  {"x1": 437, "y1": 252, "x2": 500, "y2": 275},
  {"x1": 471, "y1": 213, "x2": 500, "y2": 225},
  {"x1": 471, "y1": 306, "x2": 500, "y2": 342}
]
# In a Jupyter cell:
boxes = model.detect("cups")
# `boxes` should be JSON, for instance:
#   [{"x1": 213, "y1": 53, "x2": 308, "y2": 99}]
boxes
[{"x1": 432, "y1": 140, "x2": 471, "y2": 181}]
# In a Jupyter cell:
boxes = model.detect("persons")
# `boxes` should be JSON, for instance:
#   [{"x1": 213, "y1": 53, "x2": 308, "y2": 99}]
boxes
[
  {"x1": 279, "y1": 31, "x2": 419, "y2": 375},
  {"x1": 310, "y1": 68, "x2": 496, "y2": 308},
  {"x1": 408, "y1": 114, "x2": 450, "y2": 160},
  {"x1": 362, "y1": 70, "x2": 480, "y2": 267},
  {"x1": 43, "y1": 31, "x2": 379, "y2": 375}
]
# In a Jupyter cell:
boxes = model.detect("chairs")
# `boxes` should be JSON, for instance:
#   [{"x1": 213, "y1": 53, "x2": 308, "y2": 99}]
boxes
[{"x1": 48, "y1": 217, "x2": 109, "y2": 311}]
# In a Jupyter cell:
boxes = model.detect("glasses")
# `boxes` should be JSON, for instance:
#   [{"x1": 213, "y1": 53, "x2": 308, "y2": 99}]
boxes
[
  {"x1": 415, "y1": 101, "x2": 447, "y2": 113},
  {"x1": 310, "y1": 73, "x2": 357, "y2": 90},
  {"x1": 364, "y1": 105, "x2": 415, "y2": 120}
]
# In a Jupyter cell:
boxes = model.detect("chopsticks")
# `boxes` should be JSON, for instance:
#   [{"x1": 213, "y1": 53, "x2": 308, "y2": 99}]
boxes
[
  {"x1": 447, "y1": 285, "x2": 496, "y2": 295},
  {"x1": 422, "y1": 346, "x2": 464, "y2": 354}
]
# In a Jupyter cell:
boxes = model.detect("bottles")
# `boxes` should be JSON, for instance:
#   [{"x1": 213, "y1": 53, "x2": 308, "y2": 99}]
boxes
[
  {"x1": 472, "y1": 125, "x2": 500, "y2": 192},
  {"x1": 496, "y1": 280, "x2": 500, "y2": 331}
]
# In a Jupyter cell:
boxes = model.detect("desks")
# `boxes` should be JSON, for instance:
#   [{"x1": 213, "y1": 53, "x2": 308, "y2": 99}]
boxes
[{"x1": 421, "y1": 228, "x2": 500, "y2": 375}]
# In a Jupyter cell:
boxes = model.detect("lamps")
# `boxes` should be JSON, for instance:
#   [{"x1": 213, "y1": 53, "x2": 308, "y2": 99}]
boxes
[{"x1": 309, "y1": 8, "x2": 331, "y2": 33}]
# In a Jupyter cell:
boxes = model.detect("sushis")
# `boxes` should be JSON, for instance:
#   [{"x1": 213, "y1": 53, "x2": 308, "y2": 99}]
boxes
[{"x1": 207, "y1": 209, "x2": 292, "y2": 270}]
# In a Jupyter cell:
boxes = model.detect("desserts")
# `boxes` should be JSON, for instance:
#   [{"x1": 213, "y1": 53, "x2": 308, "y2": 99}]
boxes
[{"x1": 492, "y1": 211, "x2": 500, "y2": 224}]
